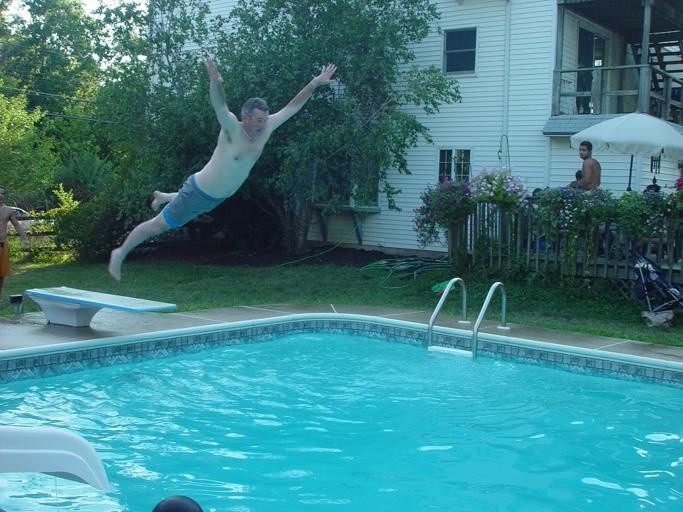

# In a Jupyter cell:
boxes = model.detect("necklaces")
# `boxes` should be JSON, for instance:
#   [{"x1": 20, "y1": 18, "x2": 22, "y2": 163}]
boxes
[{"x1": 239, "y1": 125, "x2": 257, "y2": 143}]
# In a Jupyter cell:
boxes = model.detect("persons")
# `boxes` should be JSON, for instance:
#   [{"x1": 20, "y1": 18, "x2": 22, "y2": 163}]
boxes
[
  {"x1": 568, "y1": 141, "x2": 601, "y2": 189},
  {"x1": 108, "y1": 57, "x2": 337, "y2": 280},
  {"x1": 0, "y1": 185, "x2": 28, "y2": 294},
  {"x1": 574, "y1": 169, "x2": 582, "y2": 182}
]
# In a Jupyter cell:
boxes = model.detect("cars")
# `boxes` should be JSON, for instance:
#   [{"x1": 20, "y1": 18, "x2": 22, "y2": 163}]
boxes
[{"x1": 6, "y1": 206, "x2": 44, "y2": 237}]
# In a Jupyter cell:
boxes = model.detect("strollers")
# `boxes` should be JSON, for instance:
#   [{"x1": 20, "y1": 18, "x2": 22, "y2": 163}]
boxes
[{"x1": 624, "y1": 247, "x2": 682, "y2": 330}]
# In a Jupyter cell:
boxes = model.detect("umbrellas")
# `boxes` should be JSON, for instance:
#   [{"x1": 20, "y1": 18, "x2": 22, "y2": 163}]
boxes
[{"x1": 569, "y1": 110, "x2": 682, "y2": 192}]
